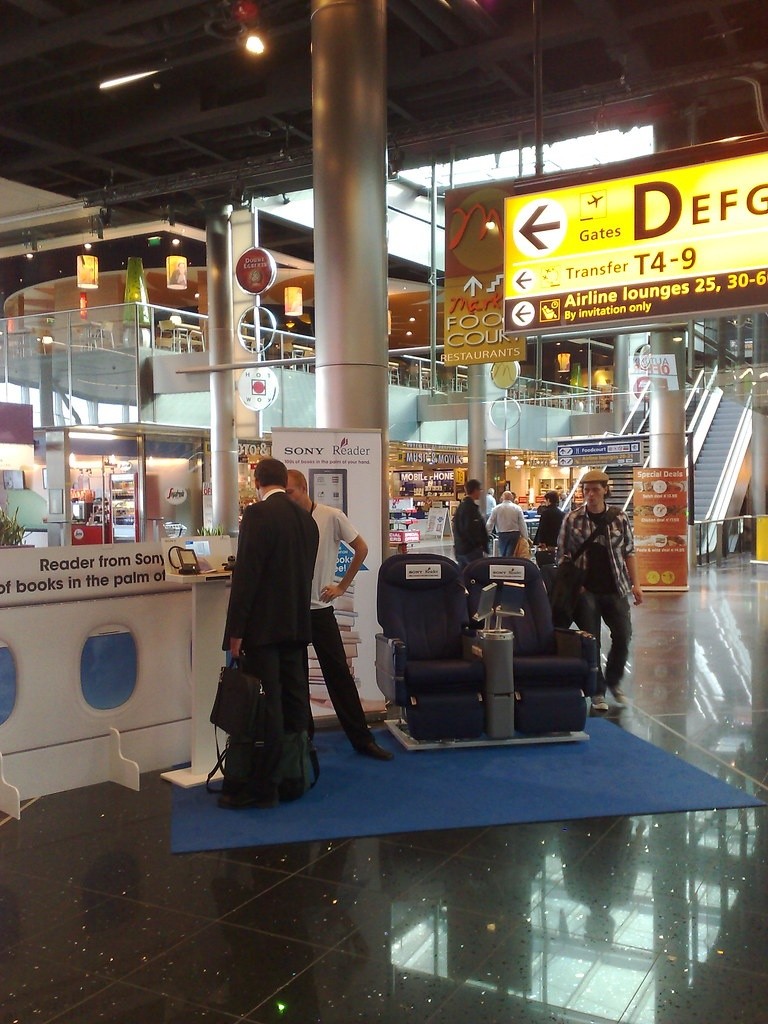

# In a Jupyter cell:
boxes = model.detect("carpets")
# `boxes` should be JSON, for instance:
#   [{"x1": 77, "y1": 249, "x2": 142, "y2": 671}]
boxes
[{"x1": 168, "y1": 724, "x2": 767, "y2": 854}]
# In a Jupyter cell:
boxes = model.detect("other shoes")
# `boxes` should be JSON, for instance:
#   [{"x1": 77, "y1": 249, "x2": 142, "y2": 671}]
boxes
[{"x1": 218, "y1": 790, "x2": 272, "y2": 810}]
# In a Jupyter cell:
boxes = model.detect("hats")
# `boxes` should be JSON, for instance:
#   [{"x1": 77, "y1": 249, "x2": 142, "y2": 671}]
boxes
[{"x1": 580, "y1": 470, "x2": 609, "y2": 484}]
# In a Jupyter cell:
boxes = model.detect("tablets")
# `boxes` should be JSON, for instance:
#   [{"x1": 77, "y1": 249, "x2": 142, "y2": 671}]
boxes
[
  {"x1": 308, "y1": 468, "x2": 347, "y2": 516},
  {"x1": 176, "y1": 548, "x2": 201, "y2": 570}
]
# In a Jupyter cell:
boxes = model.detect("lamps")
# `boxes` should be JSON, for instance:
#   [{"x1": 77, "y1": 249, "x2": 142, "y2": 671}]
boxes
[
  {"x1": 166, "y1": 203, "x2": 175, "y2": 227},
  {"x1": 95, "y1": 216, "x2": 107, "y2": 238},
  {"x1": 166, "y1": 255, "x2": 187, "y2": 290},
  {"x1": 249, "y1": 194, "x2": 255, "y2": 214},
  {"x1": 389, "y1": 156, "x2": 400, "y2": 173},
  {"x1": 557, "y1": 353, "x2": 571, "y2": 372},
  {"x1": 77, "y1": 254, "x2": 98, "y2": 290},
  {"x1": 284, "y1": 286, "x2": 303, "y2": 317},
  {"x1": 244, "y1": 27, "x2": 266, "y2": 54},
  {"x1": 30, "y1": 233, "x2": 39, "y2": 250}
]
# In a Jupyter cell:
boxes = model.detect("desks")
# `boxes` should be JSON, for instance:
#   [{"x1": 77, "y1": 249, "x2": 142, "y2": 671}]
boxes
[
  {"x1": 175, "y1": 323, "x2": 200, "y2": 331},
  {"x1": 241, "y1": 335, "x2": 256, "y2": 347},
  {"x1": 292, "y1": 344, "x2": 313, "y2": 373}
]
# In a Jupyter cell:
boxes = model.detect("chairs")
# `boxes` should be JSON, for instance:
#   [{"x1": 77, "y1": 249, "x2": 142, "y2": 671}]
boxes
[
  {"x1": 252, "y1": 338, "x2": 267, "y2": 362},
  {"x1": 375, "y1": 553, "x2": 493, "y2": 742},
  {"x1": 158, "y1": 321, "x2": 206, "y2": 353},
  {"x1": 463, "y1": 557, "x2": 600, "y2": 737},
  {"x1": 283, "y1": 342, "x2": 317, "y2": 373},
  {"x1": 95, "y1": 322, "x2": 116, "y2": 348},
  {"x1": 408, "y1": 364, "x2": 431, "y2": 389},
  {"x1": 389, "y1": 362, "x2": 400, "y2": 385}
]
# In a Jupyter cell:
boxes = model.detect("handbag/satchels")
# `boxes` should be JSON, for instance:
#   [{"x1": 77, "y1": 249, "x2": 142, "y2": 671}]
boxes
[
  {"x1": 552, "y1": 566, "x2": 580, "y2": 629},
  {"x1": 209, "y1": 649, "x2": 264, "y2": 741},
  {"x1": 258, "y1": 732, "x2": 321, "y2": 804}
]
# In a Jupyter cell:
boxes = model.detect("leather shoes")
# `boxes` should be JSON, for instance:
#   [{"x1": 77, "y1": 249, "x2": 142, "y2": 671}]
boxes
[{"x1": 357, "y1": 740, "x2": 394, "y2": 761}]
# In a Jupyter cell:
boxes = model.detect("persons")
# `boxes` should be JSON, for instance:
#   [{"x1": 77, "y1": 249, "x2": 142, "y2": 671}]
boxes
[
  {"x1": 453, "y1": 479, "x2": 490, "y2": 573},
  {"x1": 533, "y1": 491, "x2": 566, "y2": 565},
  {"x1": 485, "y1": 487, "x2": 529, "y2": 557},
  {"x1": 217, "y1": 458, "x2": 320, "y2": 811},
  {"x1": 556, "y1": 471, "x2": 644, "y2": 710},
  {"x1": 284, "y1": 469, "x2": 395, "y2": 762}
]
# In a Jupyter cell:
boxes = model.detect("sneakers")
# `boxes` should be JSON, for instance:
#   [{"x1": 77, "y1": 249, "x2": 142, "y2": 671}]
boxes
[
  {"x1": 591, "y1": 694, "x2": 609, "y2": 710},
  {"x1": 607, "y1": 684, "x2": 626, "y2": 704}
]
